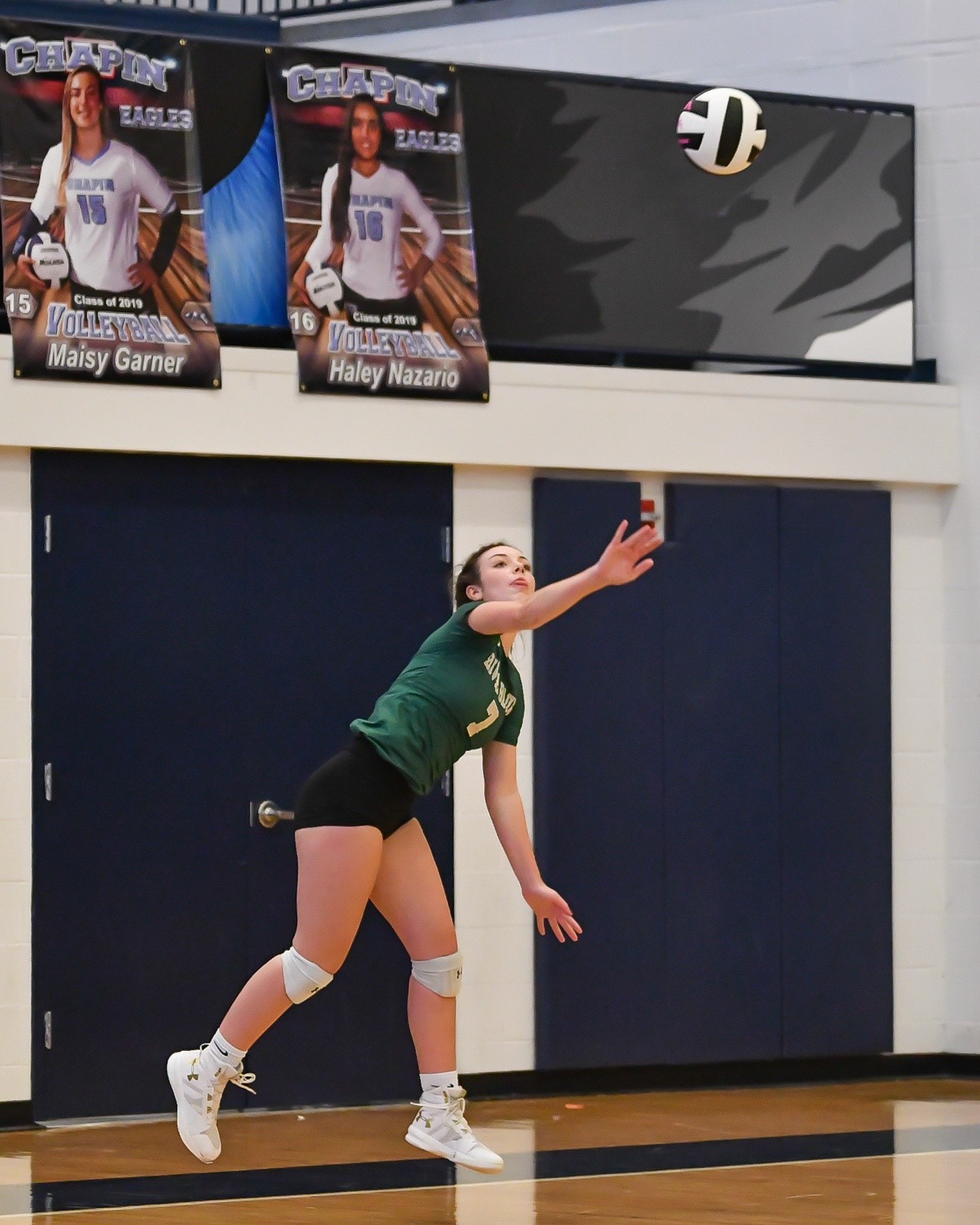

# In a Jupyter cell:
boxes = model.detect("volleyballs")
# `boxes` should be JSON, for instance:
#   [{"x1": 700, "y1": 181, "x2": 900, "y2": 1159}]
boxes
[
  {"x1": 23, "y1": 233, "x2": 73, "y2": 290},
  {"x1": 304, "y1": 262, "x2": 347, "y2": 316},
  {"x1": 676, "y1": 88, "x2": 768, "y2": 176}
]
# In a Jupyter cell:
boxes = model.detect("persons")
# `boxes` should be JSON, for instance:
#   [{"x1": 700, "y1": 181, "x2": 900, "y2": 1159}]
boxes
[
  {"x1": 166, "y1": 519, "x2": 666, "y2": 1173},
  {"x1": 13, "y1": 62, "x2": 187, "y2": 358},
  {"x1": 293, "y1": 92, "x2": 445, "y2": 348}
]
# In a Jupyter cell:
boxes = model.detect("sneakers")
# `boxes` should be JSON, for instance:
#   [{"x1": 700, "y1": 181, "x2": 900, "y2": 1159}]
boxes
[
  {"x1": 405, "y1": 1086, "x2": 505, "y2": 1174},
  {"x1": 166, "y1": 1042, "x2": 257, "y2": 1164}
]
